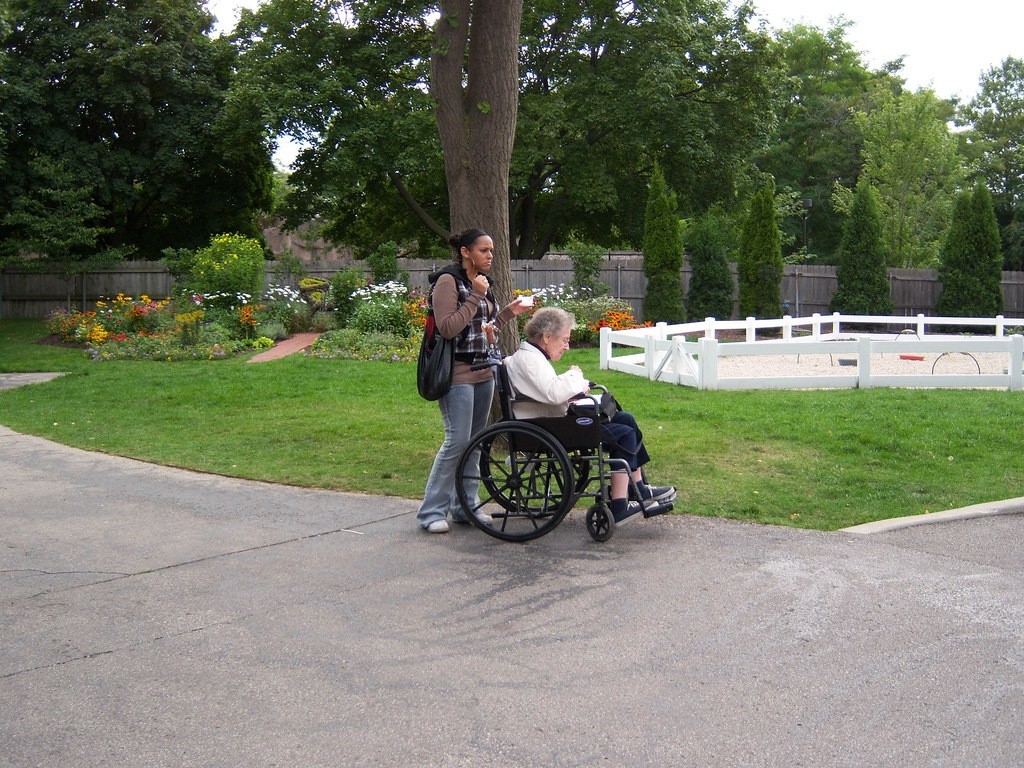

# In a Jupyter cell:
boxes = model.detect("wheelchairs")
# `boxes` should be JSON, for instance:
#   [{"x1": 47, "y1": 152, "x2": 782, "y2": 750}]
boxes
[{"x1": 455, "y1": 346, "x2": 677, "y2": 542}]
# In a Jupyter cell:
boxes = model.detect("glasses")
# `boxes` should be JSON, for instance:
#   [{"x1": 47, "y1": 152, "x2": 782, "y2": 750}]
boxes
[{"x1": 566, "y1": 337, "x2": 572, "y2": 343}]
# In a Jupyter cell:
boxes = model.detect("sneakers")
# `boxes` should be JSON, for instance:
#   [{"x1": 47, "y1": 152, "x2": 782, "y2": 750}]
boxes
[
  {"x1": 428, "y1": 519, "x2": 449, "y2": 534},
  {"x1": 452, "y1": 508, "x2": 493, "y2": 526},
  {"x1": 612, "y1": 500, "x2": 658, "y2": 527},
  {"x1": 636, "y1": 484, "x2": 674, "y2": 499}
]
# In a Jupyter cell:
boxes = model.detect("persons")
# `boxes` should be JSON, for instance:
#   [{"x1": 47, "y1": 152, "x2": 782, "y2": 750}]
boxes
[
  {"x1": 416, "y1": 229, "x2": 536, "y2": 533},
  {"x1": 503, "y1": 307, "x2": 677, "y2": 527}
]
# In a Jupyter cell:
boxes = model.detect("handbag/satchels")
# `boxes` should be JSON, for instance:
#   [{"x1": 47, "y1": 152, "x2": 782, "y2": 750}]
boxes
[
  {"x1": 416, "y1": 332, "x2": 454, "y2": 399},
  {"x1": 568, "y1": 392, "x2": 618, "y2": 424}
]
